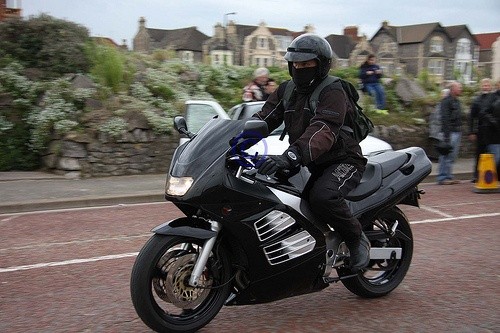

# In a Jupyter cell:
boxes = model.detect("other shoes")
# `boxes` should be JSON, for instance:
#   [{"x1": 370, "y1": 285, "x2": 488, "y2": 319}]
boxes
[
  {"x1": 376, "y1": 109, "x2": 389, "y2": 115},
  {"x1": 438, "y1": 177, "x2": 461, "y2": 186}
]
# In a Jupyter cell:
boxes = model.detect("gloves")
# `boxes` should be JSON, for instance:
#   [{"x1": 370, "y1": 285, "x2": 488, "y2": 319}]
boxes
[{"x1": 254, "y1": 147, "x2": 301, "y2": 179}]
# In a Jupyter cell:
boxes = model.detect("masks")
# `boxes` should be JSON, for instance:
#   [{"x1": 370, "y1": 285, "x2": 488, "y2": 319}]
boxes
[{"x1": 290, "y1": 65, "x2": 320, "y2": 87}]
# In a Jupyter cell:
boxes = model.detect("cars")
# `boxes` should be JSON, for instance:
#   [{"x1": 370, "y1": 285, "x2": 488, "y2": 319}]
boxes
[{"x1": 178, "y1": 100, "x2": 394, "y2": 161}]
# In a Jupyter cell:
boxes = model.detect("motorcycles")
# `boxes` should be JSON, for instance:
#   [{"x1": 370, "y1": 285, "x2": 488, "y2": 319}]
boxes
[{"x1": 130, "y1": 114, "x2": 433, "y2": 333}]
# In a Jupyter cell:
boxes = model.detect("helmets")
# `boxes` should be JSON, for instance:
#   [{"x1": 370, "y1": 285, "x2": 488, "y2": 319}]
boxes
[{"x1": 284, "y1": 33, "x2": 334, "y2": 90}]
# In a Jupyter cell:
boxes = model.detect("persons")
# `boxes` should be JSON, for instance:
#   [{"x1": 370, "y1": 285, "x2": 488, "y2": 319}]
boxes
[
  {"x1": 359, "y1": 55, "x2": 389, "y2": 109},
  {"x1": 242, "y1": 67, "x2": 279, "y2": 101},
  {"x1": 238, "y1": 33, "x2": 371, "y2": 270},
  {"x1": 468, "y1": 78, "x2": 493, "y2": 183},
  {"x1": 437, "y1": 82, "x2": 463, "y2": 185},
  {"x1": 479, "y1": 77, "x2": 500, "y2": 173}
]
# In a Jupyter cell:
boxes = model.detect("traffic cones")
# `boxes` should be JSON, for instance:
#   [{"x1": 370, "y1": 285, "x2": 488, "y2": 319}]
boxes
[{"x1": 472, "y1": 154, "x2": 500, "y2": 193}]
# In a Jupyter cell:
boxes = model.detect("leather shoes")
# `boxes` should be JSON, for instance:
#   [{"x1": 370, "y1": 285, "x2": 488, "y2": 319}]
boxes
[{"x1": 348, "y1": 231, "x2": 371, "y2": 271}]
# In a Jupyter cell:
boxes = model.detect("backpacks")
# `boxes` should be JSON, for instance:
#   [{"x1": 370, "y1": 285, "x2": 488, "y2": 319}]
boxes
[{"x1": 279, "y1": 76, "x2": 375, "y2": 145}]
